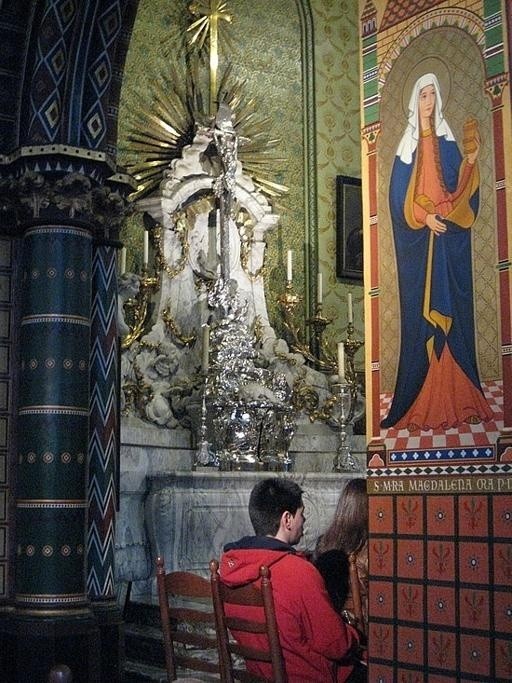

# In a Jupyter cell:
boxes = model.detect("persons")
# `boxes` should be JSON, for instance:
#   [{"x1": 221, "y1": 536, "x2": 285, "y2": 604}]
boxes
[
  {"x1": 379, "y1": 68, "x2": 495, "y2": 434},
  {"x1": 309, "y1": 477, "x2": 370, "y2": 638},
  {"x1": 216, "y1": 476, "x2": 370, "y2": 682}
]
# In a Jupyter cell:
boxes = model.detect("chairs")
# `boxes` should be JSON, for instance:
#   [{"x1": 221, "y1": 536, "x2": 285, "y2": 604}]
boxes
[
  {"x1": 153, "y1": 558, "x2": 223, "y2": 678},
  {"x1": 207, "y1": 558, "x2": 293, "y2": 679}
]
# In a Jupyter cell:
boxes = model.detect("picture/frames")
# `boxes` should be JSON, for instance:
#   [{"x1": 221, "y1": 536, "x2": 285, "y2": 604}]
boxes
[{"x1": 336, "y1": 174, "x2": 363, "y2": 278}]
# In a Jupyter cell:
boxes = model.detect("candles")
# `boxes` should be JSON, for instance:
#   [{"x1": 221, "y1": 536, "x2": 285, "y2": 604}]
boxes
[
  {"x1": 337, "y1": 342, "x2": 344, "y2": 383},
  {"x1": 286, "y1": 250, "x2": 293, "y2": 284},
  {"x1": 143, "y1": 230, "x2": 149, "y2": 269},
  {"x1": 317, "y1": 273, "x2": 323, "y2": 315},
  {"x1": 201, "y1": 323, "x2": 211, "y2": 373},
  {"x1": 347, "y1": 292, "x2": 353, "y2": 326}
]
